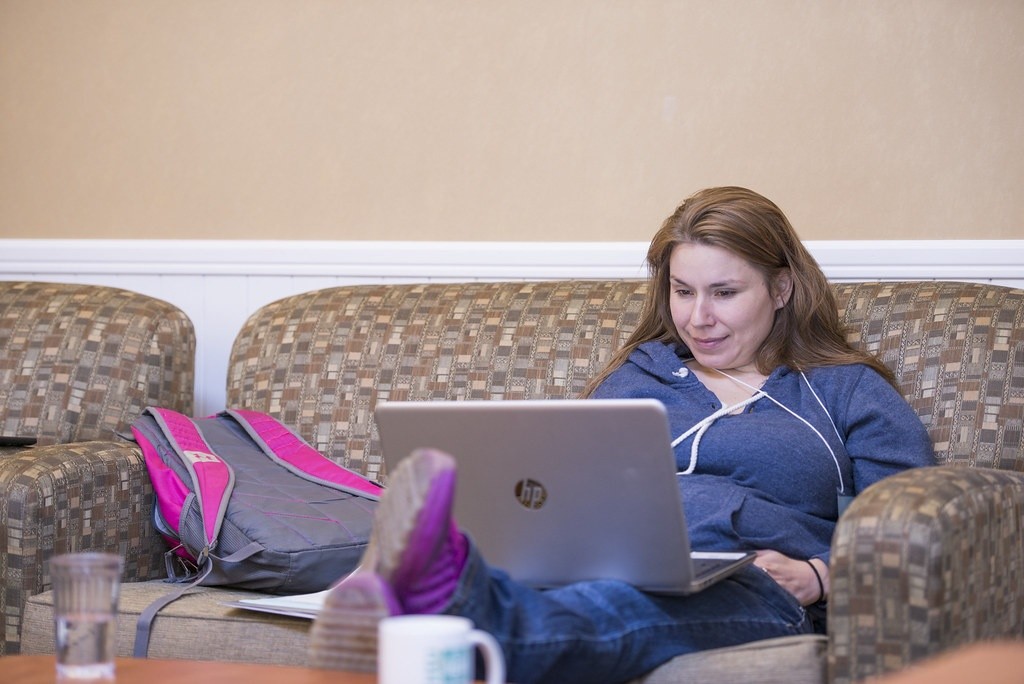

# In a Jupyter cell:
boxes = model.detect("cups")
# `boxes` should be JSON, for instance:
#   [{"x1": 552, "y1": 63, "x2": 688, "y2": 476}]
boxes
[
  {"x1": 378, "y1": 614, "x2": 506, "y2": 684},
  {"x1": 47, "y1": 553, "x2": 122, "y2": 681}
]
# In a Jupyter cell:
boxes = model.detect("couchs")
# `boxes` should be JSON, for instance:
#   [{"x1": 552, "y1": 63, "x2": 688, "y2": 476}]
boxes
[{"x1": 1, "y1": 278, "x2": 1024, "y2": 684}]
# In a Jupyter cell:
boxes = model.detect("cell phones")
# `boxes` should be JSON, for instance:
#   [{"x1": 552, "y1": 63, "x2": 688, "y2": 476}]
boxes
[{"x1": 0, "y1": 435, "x2": 37, "y2": 448}]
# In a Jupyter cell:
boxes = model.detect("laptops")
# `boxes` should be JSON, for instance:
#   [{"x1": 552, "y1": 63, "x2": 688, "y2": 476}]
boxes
[{"x1": 376, "y1": 399, "x2": 757, "y2": 592}]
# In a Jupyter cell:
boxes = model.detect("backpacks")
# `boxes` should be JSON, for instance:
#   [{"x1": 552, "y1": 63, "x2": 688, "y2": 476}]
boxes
[{"x1": 130, "y1": 405, "x2": 388, "y2": 658}]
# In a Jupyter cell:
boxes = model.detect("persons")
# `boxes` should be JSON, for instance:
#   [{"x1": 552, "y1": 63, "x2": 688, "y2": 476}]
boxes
[{"x1": 308, "y1": 184, "x2": 937, "y2": 684}]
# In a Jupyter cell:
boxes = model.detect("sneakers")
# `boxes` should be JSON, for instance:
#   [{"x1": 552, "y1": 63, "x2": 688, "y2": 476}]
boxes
[{"x1": 307, "y1": 447, "x2": 468, "y2": 671}]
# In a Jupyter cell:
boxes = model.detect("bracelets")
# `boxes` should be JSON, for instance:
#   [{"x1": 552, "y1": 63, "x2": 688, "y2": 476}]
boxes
[{"x1": 804, "y1": 559, "x2": 825, "y2": 603}]
